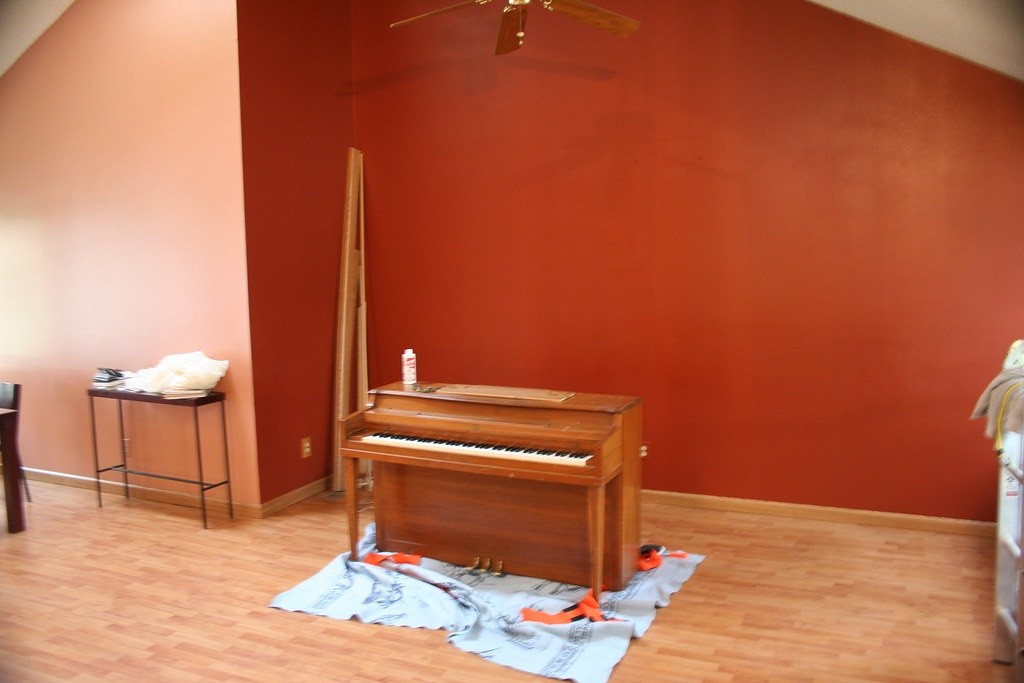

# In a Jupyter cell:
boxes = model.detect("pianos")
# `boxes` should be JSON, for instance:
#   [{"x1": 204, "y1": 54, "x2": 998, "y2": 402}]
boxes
[{"x1": 341, "y1": 378, "x2": 641, "y2": 602}]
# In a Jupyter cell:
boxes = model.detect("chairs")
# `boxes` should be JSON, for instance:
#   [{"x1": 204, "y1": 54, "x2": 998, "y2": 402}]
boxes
[{"x1": 0, "y1": 382, "x2": 33, "y2": 502}]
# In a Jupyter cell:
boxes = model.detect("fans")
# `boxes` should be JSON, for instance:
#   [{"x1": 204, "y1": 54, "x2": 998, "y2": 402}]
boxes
[{"x1": 390, "y1": 0, "x2": 639, "y2": 57}]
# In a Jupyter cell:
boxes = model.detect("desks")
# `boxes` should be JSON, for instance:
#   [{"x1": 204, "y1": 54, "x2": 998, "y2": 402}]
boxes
[{"x1": 87, "y1": 386, "x2": 236, "y2": 531}]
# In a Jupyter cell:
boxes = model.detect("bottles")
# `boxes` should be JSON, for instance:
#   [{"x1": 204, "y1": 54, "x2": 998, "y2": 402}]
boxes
[{"x1": 401, "y1": 349, "x2": 416, "y2": 384}]
